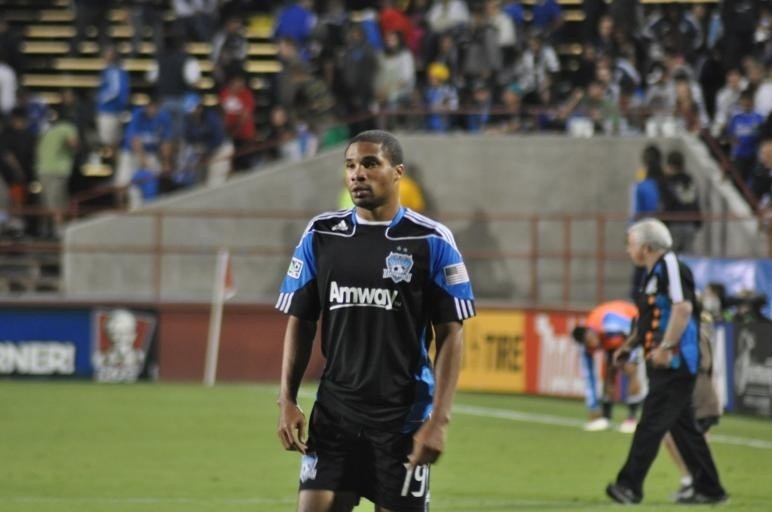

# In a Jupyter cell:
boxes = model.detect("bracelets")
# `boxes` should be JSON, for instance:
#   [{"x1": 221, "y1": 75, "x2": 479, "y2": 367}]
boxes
[{"x1": 659, "y1": 337, "x2": 676, "y2": 354}]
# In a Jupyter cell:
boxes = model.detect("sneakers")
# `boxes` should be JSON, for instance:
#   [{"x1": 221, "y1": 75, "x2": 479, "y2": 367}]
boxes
[
  {"x1": 606, "y1": 483, "x2": 641, "y2": 505},
  {"x1": 676, "y1": 476, "x2": 730, "y2": 505},
  {"x1": 583, "y1": 417, "x2": 636, "y2": 435}
]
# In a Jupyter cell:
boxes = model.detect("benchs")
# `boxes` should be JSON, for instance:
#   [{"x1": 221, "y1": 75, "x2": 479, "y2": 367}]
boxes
[{"x1": 18, "y1": 0, "x2": 305, "y2": 181}]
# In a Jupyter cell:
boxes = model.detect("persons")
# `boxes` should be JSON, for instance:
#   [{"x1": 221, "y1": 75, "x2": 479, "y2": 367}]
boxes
[
  {"x1": 665, "y1": 277, "x2": 736, "y2": 508},
  {"x1": 93, "y1": 308, "x2": 147, "y2": 387},
  {"x1": 632, "y1": 144, "x2": 703, "y2": 254},
  {"x1": 272, "y1": 127, "x2": 476, "y2": 510},
  {"x1": 727, "y1": 287, "x2": 771, "y2": 411},
  {"x1": 604, "y1": 216, "x2": 729, "y2": 507},
  {"x1": 0, "y1": 3, "x2": 771, "y2": 237},
  {"x1": 571, "y1": 298, "x2": 650, "y2": 436}
]
